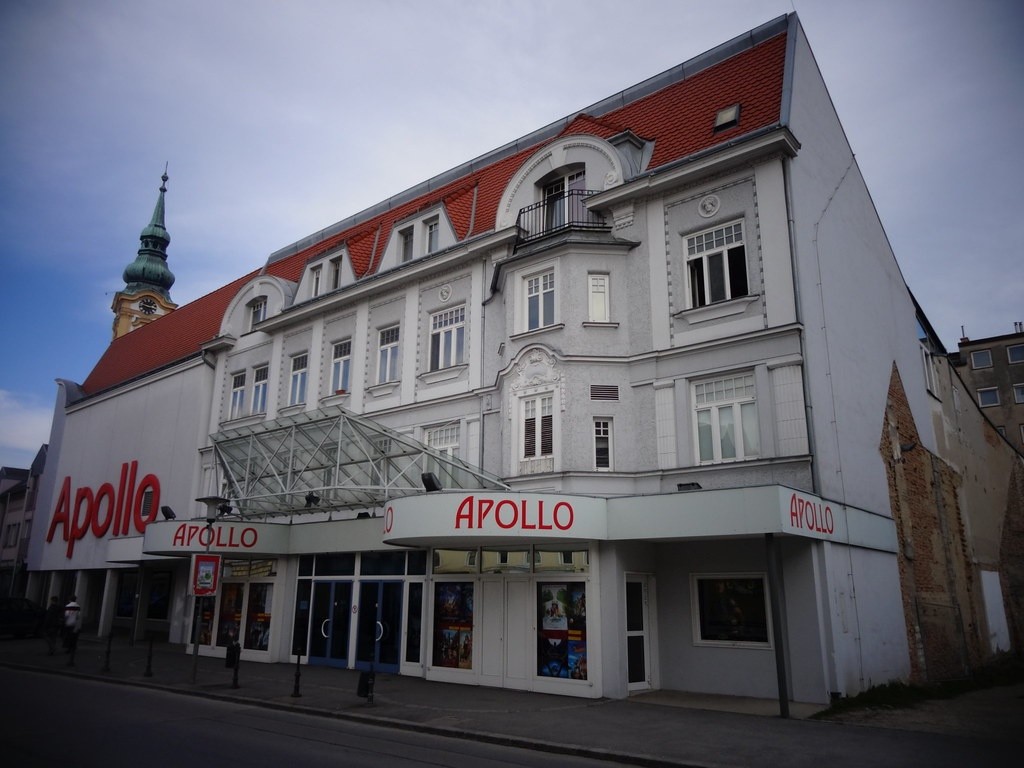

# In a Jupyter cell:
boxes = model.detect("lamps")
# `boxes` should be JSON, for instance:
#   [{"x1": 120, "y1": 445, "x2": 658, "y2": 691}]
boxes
[
  {"x1": 217, "y1": 505, "x2": 233, "y2": 516},
  {"x1": 357, "y1": 512, "x2": 371, "y2": 519},
  {"x1": 304, "y1": 495, "x2": 320, "y2": 508},
  {"x1": 161, "y1": 506, "x2": 176, "y2": 520},
  {"x1": 421, "y1": 472, "x2": 443, "y2": 493},
  {"x1": 677, "y1": 482, "x2": 702, "y2": 491}
]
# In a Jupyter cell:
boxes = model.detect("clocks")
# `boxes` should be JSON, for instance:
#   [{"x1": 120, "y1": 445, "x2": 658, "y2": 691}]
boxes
[{"x1": 139, "y1": 298, "x2": 157, "y2": 315}]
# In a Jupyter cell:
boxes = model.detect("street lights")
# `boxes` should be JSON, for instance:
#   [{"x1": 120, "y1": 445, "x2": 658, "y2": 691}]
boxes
[{"x1": 189, "y1": 494, "x2": 232, "y2": 686}]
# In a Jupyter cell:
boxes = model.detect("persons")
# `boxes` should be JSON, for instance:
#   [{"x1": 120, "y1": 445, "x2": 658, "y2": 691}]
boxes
[
  {"x1": 41, "y1": 597, "x2": 82, "y2": 655},
  {"x1": 551, "y1": 601, "x2": 562, "y2": 617},
  {"x1": 461, "y1": 635, "x2": 471, "y2": 663}
]
